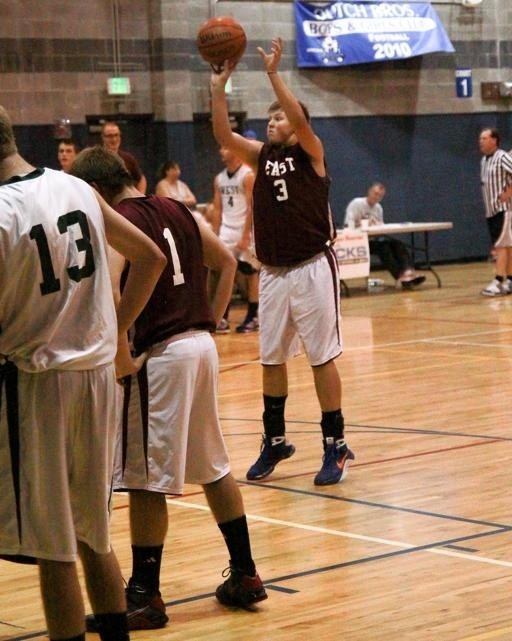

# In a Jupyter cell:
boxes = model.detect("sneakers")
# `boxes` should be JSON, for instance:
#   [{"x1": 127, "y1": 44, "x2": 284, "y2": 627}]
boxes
[
  {"x1": 402, "y1": 276, "x2": 425, "y2": 288},
  {"x1": 85, "y1": 581, "x2": 169, "y2": 631},
  {"x1": 315, "y1": 437, "x2": 354, "y2": 485},
  {"x1": 216, "y1": 317, "x2": 259, "y2": 333},
  {"x1": 482, "y1": 278, "x2": 512, "y2": 296},
  {"x1": 247, "y1": 433, "x2": 295, "y2": 480},
  {"x1": 216, "y1": 560, "x2": 267, "y2": 604}
]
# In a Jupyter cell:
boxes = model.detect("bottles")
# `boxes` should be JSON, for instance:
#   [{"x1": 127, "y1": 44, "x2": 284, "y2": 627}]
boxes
[{"x1": 368, "y1": 278, "x2": 385, "y2": 286}]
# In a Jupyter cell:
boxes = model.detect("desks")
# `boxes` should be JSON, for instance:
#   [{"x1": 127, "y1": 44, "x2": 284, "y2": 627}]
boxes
[{"x1": 334, "y1": 222, "x2": 454, "y2": 299}]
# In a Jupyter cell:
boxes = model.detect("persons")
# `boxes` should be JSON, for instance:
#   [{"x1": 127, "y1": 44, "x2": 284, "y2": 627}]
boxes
[
  {"x1": 208, "y1": 35, "x2": 354, "y2": 487},
  {"x1": 0, "y1": 107, "x2": 170, "y2": 640},
  {"x1": 72, "y1": 147, "x2": 268, "y2": 633},
  {"x1": 58, "y1": 140, "x2": 80, "y2": 173},
  {"x1": 479, "y1": 127, "x2": 512, "y2": 296},
  {"x1": 345, "y1": 183, "x2": 426, "y2": 290},
  {"x1": 156, "y1": 160, "x2": 197, "y2": 210},
  {"x1": 100, "y1": 123, "x2": 147, "y2": 198},
  {"x1": 211, "y1": 140, "x2": 261, "y2": 334}
]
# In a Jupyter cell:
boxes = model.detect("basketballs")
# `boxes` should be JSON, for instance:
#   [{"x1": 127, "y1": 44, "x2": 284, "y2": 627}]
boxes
[{"x1": 197, "y1": 14, "x2": 246, "y2": 64}]
